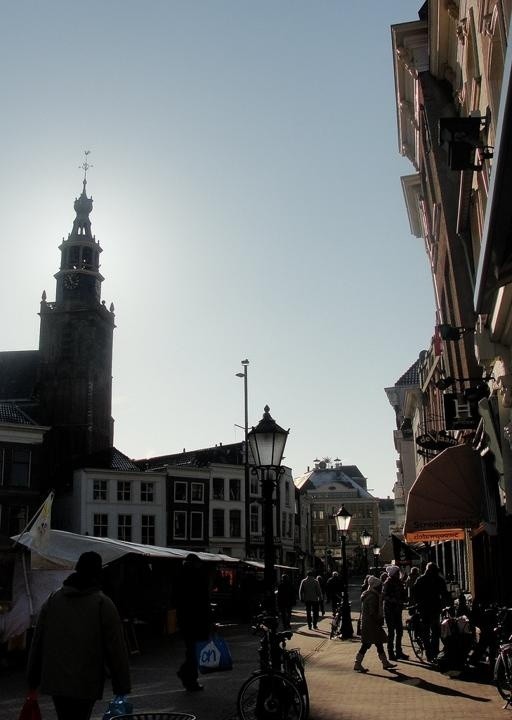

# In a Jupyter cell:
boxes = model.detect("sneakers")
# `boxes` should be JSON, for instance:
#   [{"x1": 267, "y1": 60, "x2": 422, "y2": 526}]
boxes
[{"x1": 176, "y1": 670, "x2": 203, "y2": 691}]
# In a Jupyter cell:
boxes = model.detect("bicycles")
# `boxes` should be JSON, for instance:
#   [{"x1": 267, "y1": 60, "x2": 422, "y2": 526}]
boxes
[
  {"x1": 236, "y1": 613, "x2": 311, "y2": 720},
  {"x1": 329, "y1": 600, "x2": 351, "y2": 639},
  {"x1": 481, "y1": 603, "x2": 511, "y2": 710},
  {"x1": 405, "y1": 590, "x2": 453, "y2": 659}
]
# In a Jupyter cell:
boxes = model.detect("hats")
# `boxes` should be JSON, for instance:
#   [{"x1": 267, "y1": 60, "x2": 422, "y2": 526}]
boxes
[
  {"x1": 368, "y1": 575, "x2": 381, "y2": 589},
  {"x1": 386, "y1": 565, "x2": 399, "y2": 576}
]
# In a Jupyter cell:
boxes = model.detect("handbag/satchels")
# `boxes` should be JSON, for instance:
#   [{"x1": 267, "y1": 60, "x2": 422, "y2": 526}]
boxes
[
  {"x1": 102, "y1": 701, "x2": 132, "y2": 719},
  {"x1": 195, "y1": 637, "x2": 233, "y2": 674}
]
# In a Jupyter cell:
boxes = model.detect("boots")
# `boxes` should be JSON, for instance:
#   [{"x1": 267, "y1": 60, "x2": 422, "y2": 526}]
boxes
[
  {"x1": 377, "y1": 652, "x2": 397, "y2": 669},
  {"x1": 353, "y1": 652, "x2": 369, "y2": 671},
  {"x1": 388, "y1": 649, "x2": 409, "y2": 660}
]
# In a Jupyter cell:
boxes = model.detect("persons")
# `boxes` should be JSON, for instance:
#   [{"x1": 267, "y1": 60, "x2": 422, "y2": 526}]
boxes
[
  {"x1": 274, "y1": 569, "x2": 341, "y2": 632},
  {"x1": 23, "y1": 549, "x2": 132, "y2": 720},
  {"x1": 353, "y1": 563, "x2": 467, "y2": 674},
  {"x1": 169, "y1": 554, "x2": 211, "y2": 693}
]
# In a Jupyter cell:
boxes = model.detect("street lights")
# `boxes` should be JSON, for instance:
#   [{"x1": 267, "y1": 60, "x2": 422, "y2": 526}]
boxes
[
  {"x1": 373, "y1": 544, "x2": 380, "y2": 578},
  {"x1": 360, "y1": 528, "x2": 371, "y2": 577},
  {"x1": 332, "y1": 504, "x2": 353, "y2": 638},
  {"x1": 247, "y1": 407, "x2": 291, "y2": 720}
]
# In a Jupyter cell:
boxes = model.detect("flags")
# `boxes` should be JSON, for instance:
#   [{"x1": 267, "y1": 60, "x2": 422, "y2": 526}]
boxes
[{"x1": 29, "y1": 492, "x2": 52, "y2": 542}]
